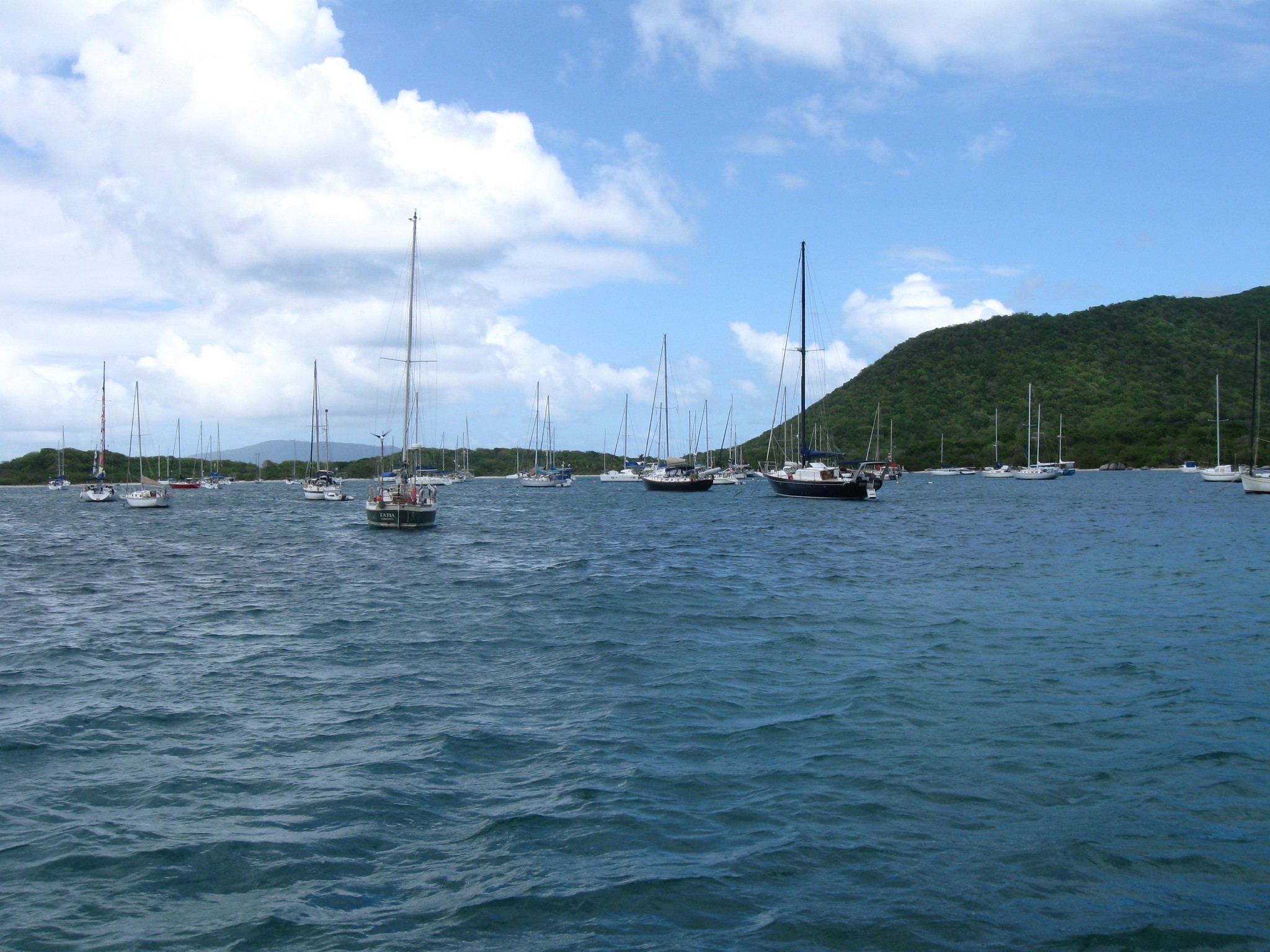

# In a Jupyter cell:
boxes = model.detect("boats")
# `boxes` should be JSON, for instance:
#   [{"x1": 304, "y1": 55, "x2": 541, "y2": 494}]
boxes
[
  {"x1": 1141, "y1": 466, "x2": 1149, "y2": 470},
  {"x1": 1127, "y1": 467, "x2": 1133, "y2": 470},
  {"x1": 931, "y1": 433, "x2": 976, "y2": 475},
  {"x1": 1238, "y1": 464, "x2": 1270, "y2": 494},
  {"x1": 1179, "y1": 461, "x2": 1204, "y2": 473}
]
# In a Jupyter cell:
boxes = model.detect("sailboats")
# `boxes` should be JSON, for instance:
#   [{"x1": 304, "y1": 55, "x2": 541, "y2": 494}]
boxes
[
  {"x1": 304, "y1": 359, "x2": 354, "y2": 500},
  {"x1": 157, "y1": 418, "x2": 237, "y2": 490},
  {"x1": 80, "y1": 361, "x2": 118, "y2": 502},
  {"x1": 255, "y1": 453, "x2": 264, "y2": 483},
  {"x1": 600, "y1": 334, "x2": 764, "y2": 493},
  {"x1": 286, "y1": 440, "x2": 303, "y2": 484},
  {"x1": 515, "y1": 382, "x2": 572, "y2": 487},
  {"x1": 48, "y1": 426, "x2": 71, "y2": 490},
  {"x1": 764, "y1": 239, "x2": 902, "y2": 501},
  {"x1": 367, "y1": 208, "x2": 476, "y2": 528},
  {"x1": 124, "y1": 381, "x2": 172, "y2": 508},
  {"x1": 982, "y1": 383, "x2": 1075, "y2": 479},
  {"x1": 1200, "y1": 374, "x2": 1250, "y2": 482}
]
